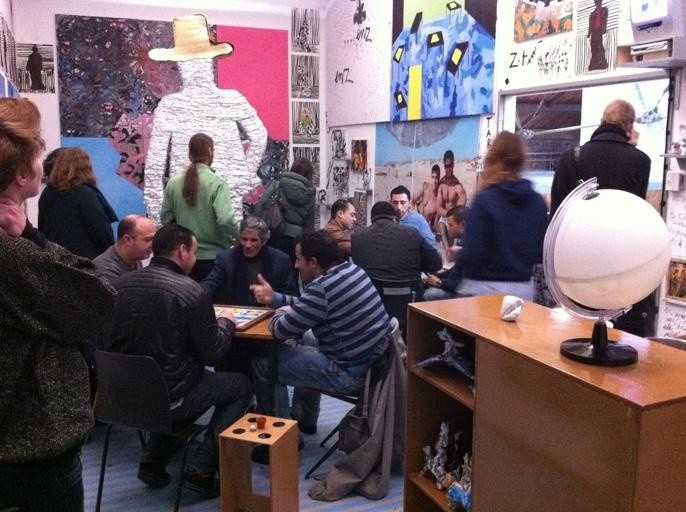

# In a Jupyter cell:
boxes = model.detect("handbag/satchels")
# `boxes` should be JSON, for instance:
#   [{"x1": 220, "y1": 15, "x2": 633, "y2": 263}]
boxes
[{"x1": 258, "y1": 180, "x2": 284, "y2": 230}]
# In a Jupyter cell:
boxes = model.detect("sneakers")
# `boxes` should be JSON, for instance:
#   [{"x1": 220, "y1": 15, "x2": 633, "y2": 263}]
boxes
[
  {"x1": 179, "y1": 475, "x2": 220, "y2": 504},
  {"x1": 251, "y1": 436, "x2": 304, "y2": 465},
  {"x1": 289, "y1": 407, "x2": 317, "y2": 434},
  {"x1": 137, "y1": 463, "x2": 170, "y2": 487}
]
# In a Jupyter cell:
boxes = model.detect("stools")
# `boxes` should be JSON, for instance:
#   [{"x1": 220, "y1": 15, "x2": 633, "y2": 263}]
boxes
[{"x1": 219, "y1": 413, "x2": 298, "y2": 512}]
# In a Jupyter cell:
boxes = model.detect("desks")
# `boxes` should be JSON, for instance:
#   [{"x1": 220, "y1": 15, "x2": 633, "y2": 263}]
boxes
[{"x1": 210, "y1": 304, "x2": 281, "y2": 416}]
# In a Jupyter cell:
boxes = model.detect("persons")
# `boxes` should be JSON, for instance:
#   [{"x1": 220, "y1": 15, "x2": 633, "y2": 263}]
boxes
[
  {"x1": 550, "y1": 101, "x2": 651, "y2": 337},
  {"x1": 94, "y1": 133, "x2": 472, "y2": 497},
  {"x1": 446, "y1": 131, "x2": 552, "y2": 301}
]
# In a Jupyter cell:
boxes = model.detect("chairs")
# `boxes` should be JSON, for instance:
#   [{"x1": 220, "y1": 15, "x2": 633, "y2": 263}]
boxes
[
  {"x1": 298, "y1": 316, "x2": 407, "y2": 502},
  {"x1": 371, "y1": 276, "x2": 422, "y2": 345},
  {"x1": 93, "y1": 348, "x2": 219, "y2": 512}
]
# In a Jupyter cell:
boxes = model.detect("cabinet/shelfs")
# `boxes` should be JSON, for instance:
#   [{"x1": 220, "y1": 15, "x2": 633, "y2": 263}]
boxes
[{"x1": 406, "y1": 293, "x2": 685, "y2": 512}]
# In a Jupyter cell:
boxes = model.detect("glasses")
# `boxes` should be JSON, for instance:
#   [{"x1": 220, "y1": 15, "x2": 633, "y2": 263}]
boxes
[{"x1": 445, "y1": 162, "x2": 454, "y2": 167}]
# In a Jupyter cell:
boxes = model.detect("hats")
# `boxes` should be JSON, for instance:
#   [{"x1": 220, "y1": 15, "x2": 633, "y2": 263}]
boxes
[{"x1": 147, "y1": 13, "x2": 234, "y2": 62}]
